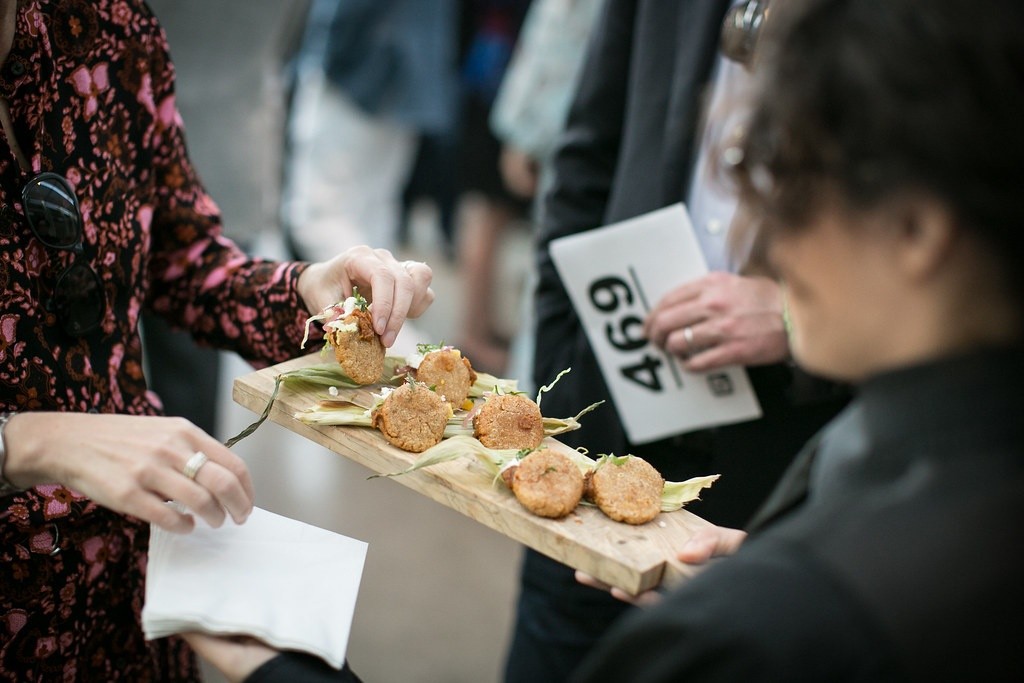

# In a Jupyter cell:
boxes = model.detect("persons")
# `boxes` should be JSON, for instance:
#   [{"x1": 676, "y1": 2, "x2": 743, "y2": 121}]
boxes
[
  {"x1": 179, "y1": 0, "x2": 1024, "y2": 683},
  {"x1": 272, "y1": 1, "x2": 606, "y2": 397},
  {"x1": 502, "y1": 0, "x2": 863, "y2": 683},
  {"x1": 0, "y1": 0, "x2": 437, "y2": 683}
]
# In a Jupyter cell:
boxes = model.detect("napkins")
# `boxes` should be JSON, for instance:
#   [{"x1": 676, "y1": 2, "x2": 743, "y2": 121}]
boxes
[{"x1": 140, "y1": 500, "x2": 370, "y2": 669}]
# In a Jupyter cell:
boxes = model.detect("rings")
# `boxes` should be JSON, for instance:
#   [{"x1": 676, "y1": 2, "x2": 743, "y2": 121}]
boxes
[
  {"x1": 684, "y1": 326, "x2": 696, "y2": 351},
  {"x1": 183, "y1": 451, "x2": 208, "y2": 478}
]
